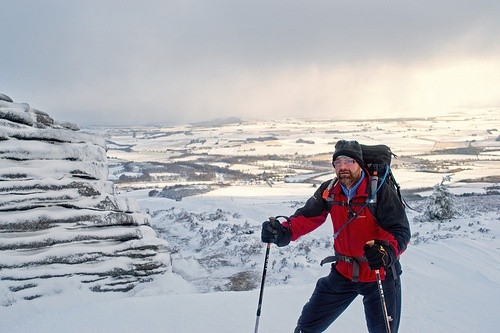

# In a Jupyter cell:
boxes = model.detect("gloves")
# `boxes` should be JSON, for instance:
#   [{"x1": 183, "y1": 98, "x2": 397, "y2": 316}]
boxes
[
  {"x1": 364, "y1": 240, "x2": 395, "y2": 272},
  {"x1": 261, "y1": 219, "x2": 291, "y2": 248}
]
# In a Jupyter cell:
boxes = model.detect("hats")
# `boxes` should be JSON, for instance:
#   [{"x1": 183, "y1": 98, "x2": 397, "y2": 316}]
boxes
[{"x1": 331, "y1": 140, "x2": 363, "y2": 168}]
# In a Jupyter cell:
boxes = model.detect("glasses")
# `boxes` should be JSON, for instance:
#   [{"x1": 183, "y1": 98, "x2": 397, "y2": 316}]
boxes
[{"x1": 331, "y1": 160, "x2": 357, "y2": 166}]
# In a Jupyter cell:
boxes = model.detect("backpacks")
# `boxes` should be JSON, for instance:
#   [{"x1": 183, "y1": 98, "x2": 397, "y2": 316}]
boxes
[{"x1": 358, "y1": 144, "x2": 406, "y2": 209}]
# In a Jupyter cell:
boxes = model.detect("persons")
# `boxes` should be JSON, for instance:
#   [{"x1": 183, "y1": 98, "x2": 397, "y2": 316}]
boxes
[{"x1": 257, "y1": 137, "x2": 408, "y2": 332}]
